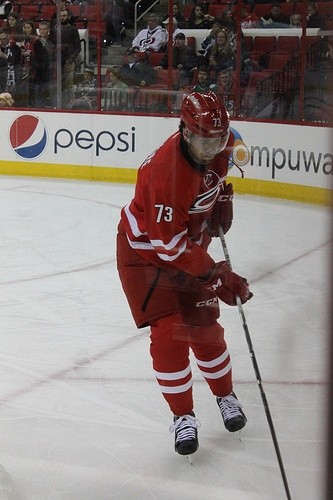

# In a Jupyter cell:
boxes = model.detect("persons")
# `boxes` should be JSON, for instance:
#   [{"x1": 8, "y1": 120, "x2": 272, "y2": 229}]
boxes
[
  {"x1": 259, "y1": 1, "x2": 303, "y2": 27},
  {"x1": 0, "y1": 0, "x2": 81, "y2": 107},
  {"x1": 217, "y1": 70, "x2": 235, "y2": 116},
  {"x1": 117, "y1": 93, "x2": 254, "y2": 464},
  {"x1": 201, "y1": 10, "x2": 251, "y2": 70},
  {"x1": 193, "y1": 66, "x2": 218, "y2": 94},
  {"x1": 305, "y1": 2, "x2": 325, "y2": 28},
  {"x1": 78, "y1": 4, "x2": 211, "y2": 114}
]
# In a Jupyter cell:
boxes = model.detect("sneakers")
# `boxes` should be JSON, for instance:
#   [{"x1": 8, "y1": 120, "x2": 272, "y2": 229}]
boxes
[
  {"x1": 216, "y1": 392, "x2": 248, "y2": 443},
  {"x1": 169, "y1": 411, "x2": 201, "y2": 466}
]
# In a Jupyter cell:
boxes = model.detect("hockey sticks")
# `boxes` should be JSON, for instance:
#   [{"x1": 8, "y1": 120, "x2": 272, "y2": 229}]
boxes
[{"x1": 219, "y1": 226, "x2": 292, "y2": 500}]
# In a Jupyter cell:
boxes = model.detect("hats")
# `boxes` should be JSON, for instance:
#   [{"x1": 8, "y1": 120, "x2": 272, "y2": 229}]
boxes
[
  {"x1": 162, "y1": 17, "x2": 177, "y2": 24},
  {"x1": 175, "y1": 33, "x2": 186, "y2": 40}
]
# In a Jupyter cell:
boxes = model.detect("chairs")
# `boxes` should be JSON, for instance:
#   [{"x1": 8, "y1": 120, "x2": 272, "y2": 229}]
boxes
[{"x1": 0, "y1": 0, "x2": 333, "y2": 122}]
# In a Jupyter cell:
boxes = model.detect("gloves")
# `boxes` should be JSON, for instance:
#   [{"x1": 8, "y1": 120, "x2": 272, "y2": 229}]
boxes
[
  {"x1": 197, "y1": 261, "x2": 254, "y2": 306},
  {"x1": 207, "y1": 183, "x2": 234, "y2": 238}
]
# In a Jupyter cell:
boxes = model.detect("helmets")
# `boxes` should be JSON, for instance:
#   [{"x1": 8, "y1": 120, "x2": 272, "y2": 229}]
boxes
[{"x1": 180, "y1": 91, "x2": 229, "y2": 136}]
